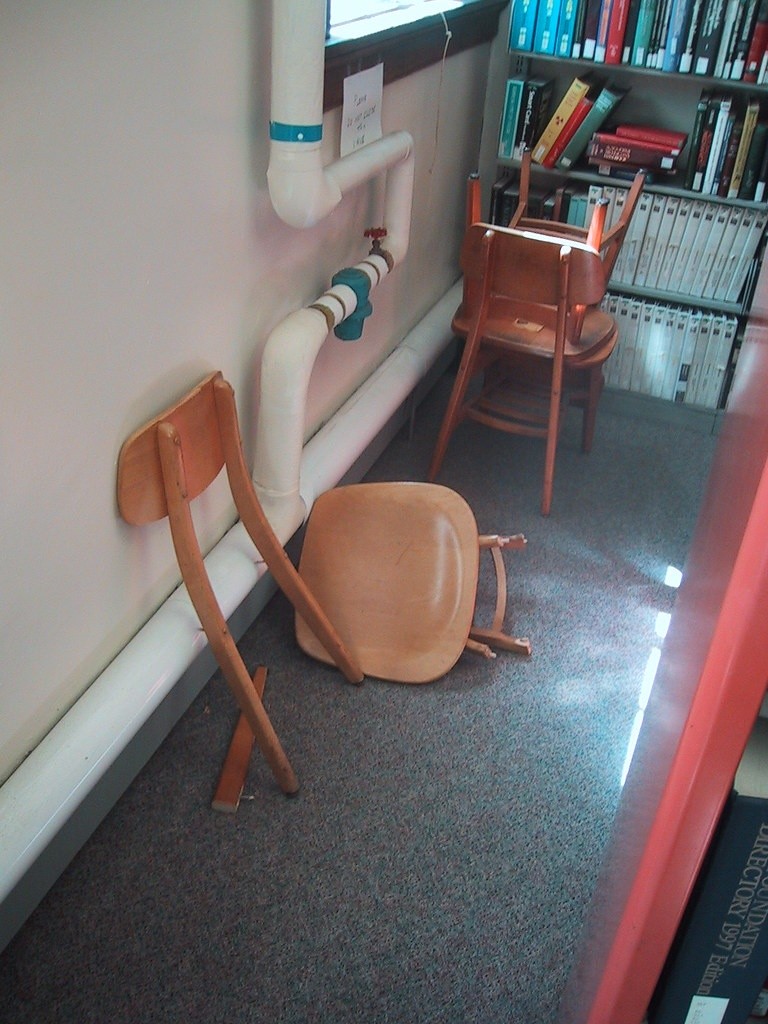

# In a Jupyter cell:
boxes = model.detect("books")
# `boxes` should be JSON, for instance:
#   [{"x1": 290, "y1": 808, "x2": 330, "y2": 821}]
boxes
[
  {"x1": 645, "y1": 716, "x2": 767, "y2": 1024},
  {"x1": 489, "y1": 177, "x2": 768, "y2": 302},
  {"x1": 511, "y1": 0, "x2": 768, "y2": 84},
  {"x1": 596, "y1": 291, "x2": 738, "y2": 410},
  {"x1": 496, "y1": 71, "x2": 768, "y2": 204}
]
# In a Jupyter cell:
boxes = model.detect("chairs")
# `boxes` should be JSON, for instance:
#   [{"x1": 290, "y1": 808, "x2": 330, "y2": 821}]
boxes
[{"x1": 427, "y1": 145, "x2": 648, "y2": 517}]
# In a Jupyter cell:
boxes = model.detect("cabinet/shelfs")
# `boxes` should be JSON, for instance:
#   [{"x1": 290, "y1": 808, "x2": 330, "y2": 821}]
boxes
[{"x1": 489, "y1": 0, "x2": 768, "y2": 409}]
